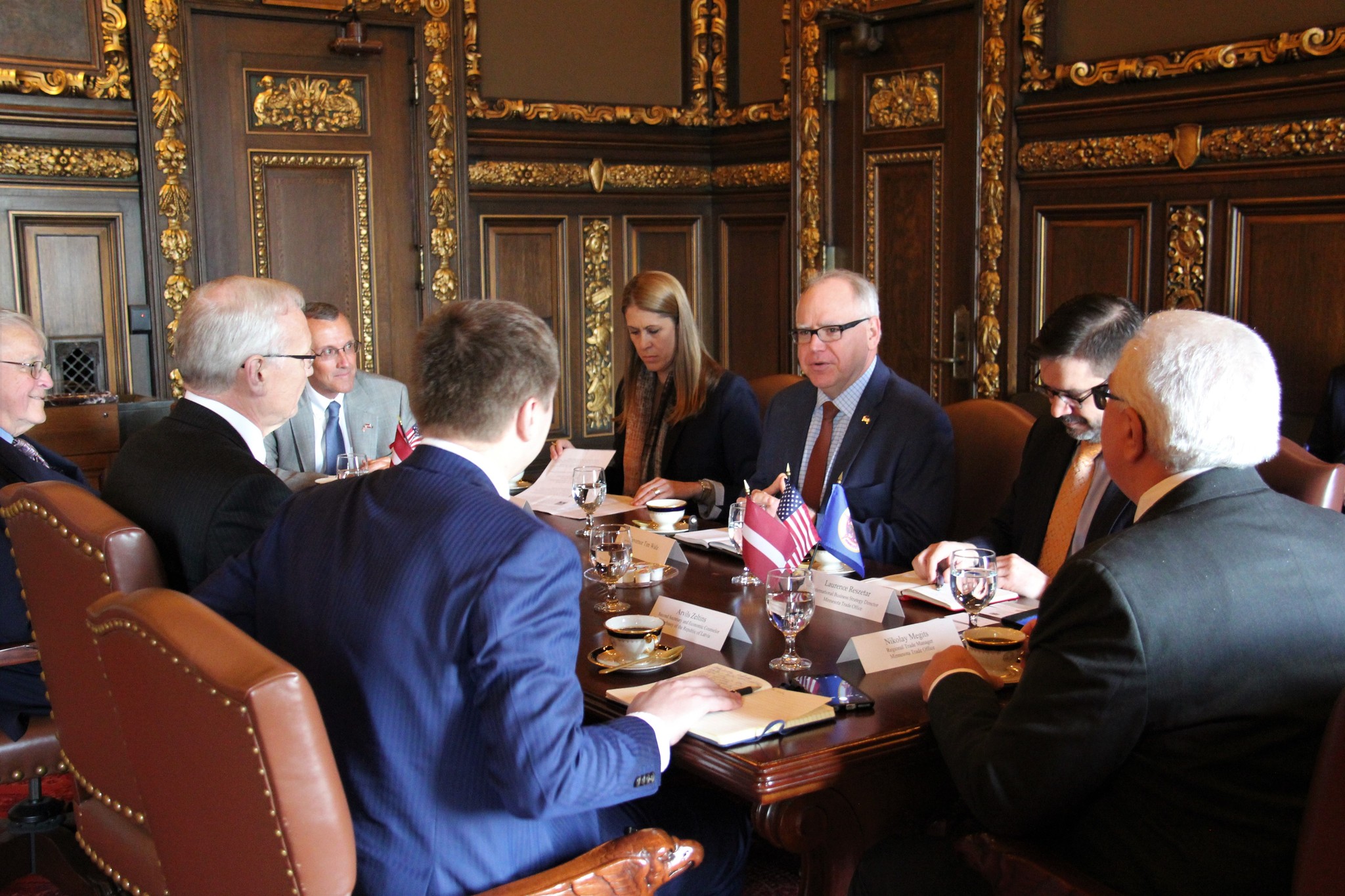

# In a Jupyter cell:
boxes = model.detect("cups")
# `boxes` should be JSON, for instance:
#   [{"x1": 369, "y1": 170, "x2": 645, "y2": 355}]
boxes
[
  {"x1": 336, "y1": 453, "x2": 369, "y2": 479},
  {"x1": 962, "y1": 627, "x2": 1027, "y2": 682},
  {"x1": 604, "y1": 614, "x2": 665, "y2": 661},
  {"x1": 646, "y1": 498, "x2": 687, "y2": 530},
  {"x1": 811, "y1": 544, "x2": 845, "y2": 572}
]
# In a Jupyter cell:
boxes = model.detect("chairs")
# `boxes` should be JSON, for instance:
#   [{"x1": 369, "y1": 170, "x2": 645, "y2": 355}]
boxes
[
  {"x1": 953, "y1": 687, "x2": 1345, "y2": 896},
  {"x1": 939, "y1": 398, "x2": 1038, "y2": 534},
  {"x1": 1257, "y1": 433, "x2": 1345, "y2": 513},
  {"x1": 0, "y1": 482, "x2": 707, "y2": 896},
  {"x1": 749, "y1": 374, "x2": 804, "y2": 421}
]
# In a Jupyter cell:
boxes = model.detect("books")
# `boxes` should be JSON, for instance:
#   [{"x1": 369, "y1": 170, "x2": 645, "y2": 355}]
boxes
[
  {"x1": 606, "y1": 662, "x2": 836, "y2": 747},
  {"x1": 670, "y1": 527, "x2": 744, "y2": 559},
  {"x1": 861, "y1": 566, "x2": 1019, "y2": 612}
]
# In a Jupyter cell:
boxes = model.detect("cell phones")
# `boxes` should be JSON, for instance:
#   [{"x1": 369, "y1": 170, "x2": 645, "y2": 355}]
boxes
[
  {"x1": 788, "y1": 673, "x2": 873, "y2": 712},
  {"x1": 509, "y1": 481, "x2": 533, "y2": 495},
  {"x1": 1000, "y1": 607, "x2": 1041, "y2": 630}
]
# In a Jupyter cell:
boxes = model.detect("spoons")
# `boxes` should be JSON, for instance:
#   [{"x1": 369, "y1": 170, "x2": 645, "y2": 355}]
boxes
[{"x1": 598, "y1": 645, "x2": 685, "y2": 674}]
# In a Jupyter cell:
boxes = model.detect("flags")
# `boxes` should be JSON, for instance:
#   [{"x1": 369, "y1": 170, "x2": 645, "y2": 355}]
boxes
[
  {"x1": 742, "y1": 494, "x2": 797, "y2": 589},
  {"x1": 774, "y1": 480, "x2": 822, "y2": 574},
  {"x1": 818, "y1": 484, "x2": 865, "y2": 579},
  {"x1": 388, "y1": 422, "x2": 424, "y2": 452},
  {"x1": 390, "y1": 424, "x2": 414, "y2": 469}
]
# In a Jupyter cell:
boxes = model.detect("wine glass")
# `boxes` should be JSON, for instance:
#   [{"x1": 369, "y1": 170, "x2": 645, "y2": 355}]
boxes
[
  {"x1": 572, "y1": 466, "x2": 607, "y2": 538},
  {"x1": 728, "y1": 502, "x2": 768, "y2": 587},
  {"x1": 590, "y1": 524, "x2": 633, "y2": 613},
  {"x1": 765, "y1": 567, "x2": 816, "y2": 671},
  {"x1": 950, "y1": 548, "x2": 997, "y2": 639}
]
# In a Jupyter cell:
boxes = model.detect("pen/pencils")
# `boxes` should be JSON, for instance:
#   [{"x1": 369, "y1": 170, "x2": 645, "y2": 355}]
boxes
[
  {"x1": 551, "y1": 442, "x2": 565, "y2": 449},
  {"x1": 731, "y1": 685, "x2": 762, "y2": 696},
  {"x1": 936, "y1": 569, "x2": 943, "y2": 589}
]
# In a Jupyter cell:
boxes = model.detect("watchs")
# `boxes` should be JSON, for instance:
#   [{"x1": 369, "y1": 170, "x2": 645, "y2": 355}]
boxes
[{"x1": 697, "y1": 479, "x2": 712, "y2": 501}]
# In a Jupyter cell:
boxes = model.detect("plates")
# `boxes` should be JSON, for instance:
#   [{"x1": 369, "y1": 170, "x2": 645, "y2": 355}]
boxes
[
  {"x1": 640, "y1": 522, "x2": 690, "y2": 535},
  {"x1": 587, "y1": 643, "x2": 682, "y2": 674},
  {"x1": 584, "y1": 562, "x2": 679, "y2": 588},
  {"x1": 799, "y1": 562, "x2": 856, "y2": 576},
  {"x1": 315, "y1": 477, "x2": 337, "y2": 485}
]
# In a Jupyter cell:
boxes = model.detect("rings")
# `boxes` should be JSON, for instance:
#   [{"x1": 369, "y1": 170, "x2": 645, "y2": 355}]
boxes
[{"x1": 653, "y1": 488, "x2": 660, "y2": 495}]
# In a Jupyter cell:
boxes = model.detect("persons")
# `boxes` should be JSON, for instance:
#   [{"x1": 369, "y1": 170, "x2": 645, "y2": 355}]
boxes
[
  {"x1": 735, "y1": 270, "x2": 959, "y2": 575},
  {"x1": 262, "y1": 302, "x2": 420, "y2": 496},
  {"x1": 0, "y1": 308, "x2": 104, "y2": 739},
  {"x1": 916, "y1": 308, "x2": 1345, "y2": 896},
  {"x1": 189, "y1": 297, "x2": 754, "y2": 896},
  {"x1": 101, "y1": 276, "x2": 315, "y2": 597},
  {"x1": 552, "y1": 270, "x2": 763, "y2": 520},
  {"x1": 912, "y1": 293, "x2": 1145, "y2": 604}
]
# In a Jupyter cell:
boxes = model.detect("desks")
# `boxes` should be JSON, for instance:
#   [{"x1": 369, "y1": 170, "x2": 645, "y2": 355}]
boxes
[{"x1": 533, "y1": 509, "x2": 1017, "y2": 896}]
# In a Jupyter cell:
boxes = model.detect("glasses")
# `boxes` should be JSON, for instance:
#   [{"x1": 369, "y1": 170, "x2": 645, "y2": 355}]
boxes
[
  {"x1": 1027, "y1": 369, "x2": 1108, "y2": 409},
  {"x1": 1091, "y1": 384, "x2": 1148, "y2": 435},
  {"x1": 0, "y1": 360, "x2": 52, "y2": 380},
  {"x1": 791, "y1": 318, "x2": 870, "y2": 344},
  {"x1": 317, "y1": 339, "x2": 362, "y2": 361},
  {"x1": 241, "y1": 350, "x2": 316, "y2": 369}
]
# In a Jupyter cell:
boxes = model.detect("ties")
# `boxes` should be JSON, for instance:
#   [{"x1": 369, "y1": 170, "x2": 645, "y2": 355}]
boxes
[
  {"x1": 801, "y1": 401, "x2": 840, "y2": 513},
  {"x1": 324, "y1": 401, "x2": 349, "y2": 476},
  {"x1": 1037, "y1": 439, "x2": 1102, "y2": 583},
  {"x1": 12, "y1": 437, "x2": 49, "y2": 469}
]
